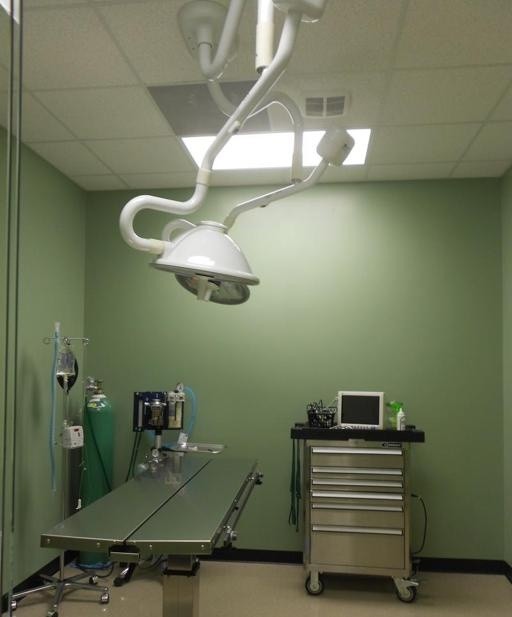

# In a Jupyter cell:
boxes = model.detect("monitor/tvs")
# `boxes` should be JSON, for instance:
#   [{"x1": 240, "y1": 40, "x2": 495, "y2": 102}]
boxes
[{"x1": 338, "y1": 391, "x2": 383, "y2": 430}]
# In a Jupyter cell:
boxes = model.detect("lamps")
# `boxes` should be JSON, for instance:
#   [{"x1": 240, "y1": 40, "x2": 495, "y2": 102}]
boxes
[{"x1": 122, "y1": 0, "x2": 352, "y2": 306}]
